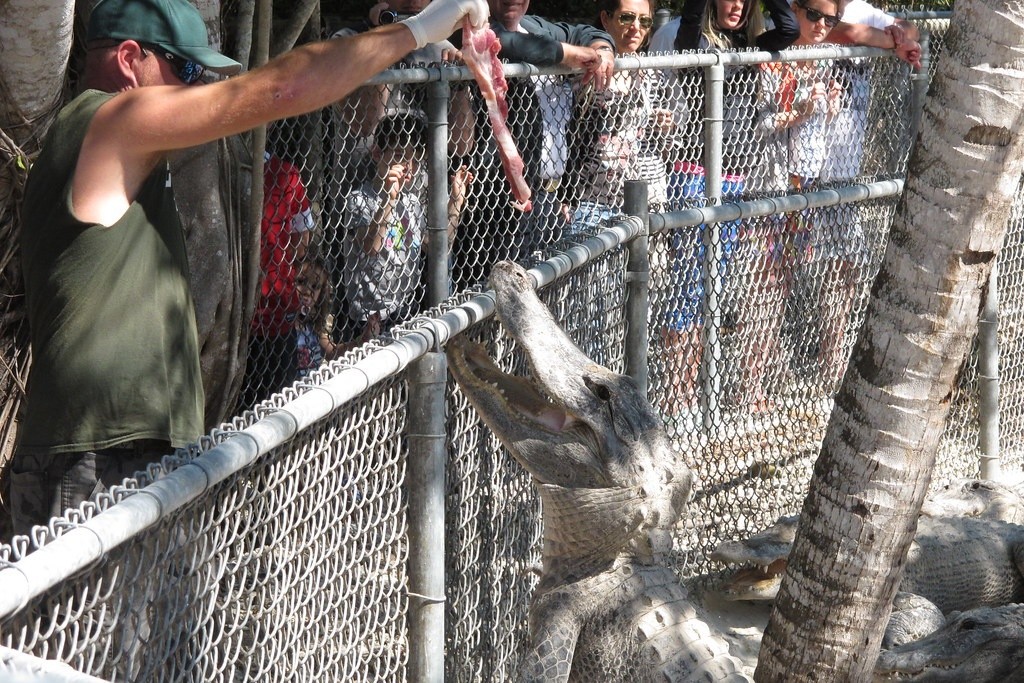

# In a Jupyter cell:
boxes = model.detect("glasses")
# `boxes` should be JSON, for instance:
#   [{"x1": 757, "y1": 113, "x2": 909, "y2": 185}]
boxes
[
  {"x1": 798, "y1": 4, "x2": 838, "y2": 27},
  {"x1": 123, "y1": 39, "x2": 206, "y2": 85},
  {"x1": 607, "y1": 12, "x2": 654, "y2": 29}
]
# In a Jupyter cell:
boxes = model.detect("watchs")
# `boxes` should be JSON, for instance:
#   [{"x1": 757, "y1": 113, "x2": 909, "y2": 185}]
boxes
[{"x1": 597, "y1": 45, "x2": 613, "y2": 53}]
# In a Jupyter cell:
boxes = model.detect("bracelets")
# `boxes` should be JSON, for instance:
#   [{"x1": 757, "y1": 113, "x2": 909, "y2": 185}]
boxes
[{"x1": 450, "y1": 81, "x2": 467, "y2": 91}]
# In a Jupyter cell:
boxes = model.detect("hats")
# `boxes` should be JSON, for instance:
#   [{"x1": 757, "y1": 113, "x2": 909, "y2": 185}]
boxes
[{"x1": 88, "y1": 0, "x2": 242, "y2": 76}]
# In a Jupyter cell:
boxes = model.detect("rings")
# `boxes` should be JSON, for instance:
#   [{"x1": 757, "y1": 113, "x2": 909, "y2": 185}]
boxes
[{"x1": 601, "y1": 71, "x2": 606, "y2": 74}]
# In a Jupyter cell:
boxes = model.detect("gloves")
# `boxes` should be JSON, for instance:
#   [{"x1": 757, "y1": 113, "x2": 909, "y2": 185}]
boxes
[{"x1": 396, "y1": 0, "x2": 490, "y2": 51}]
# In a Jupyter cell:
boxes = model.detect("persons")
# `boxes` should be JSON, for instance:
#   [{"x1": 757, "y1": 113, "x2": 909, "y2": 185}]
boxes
[
  {"x1": 7, "y1": 0, "x2": 493, "y2": 683},
  {"x1": 231, "y1": 1, "x2": 922, "y2": 543}
]
